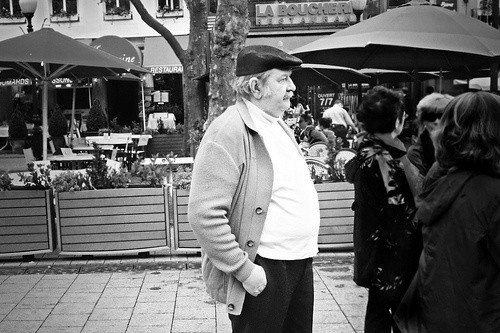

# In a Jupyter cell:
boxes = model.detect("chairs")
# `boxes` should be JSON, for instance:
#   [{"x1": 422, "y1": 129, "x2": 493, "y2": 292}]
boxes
[
  {"x1": 0, "y1": 127, "x2": 151, "y2": 171},
  {"x1": 298, "y1": 124, "x2": 368, "y2": 185}
]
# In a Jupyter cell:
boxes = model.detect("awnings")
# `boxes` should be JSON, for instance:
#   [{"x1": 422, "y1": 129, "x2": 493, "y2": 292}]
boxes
[{"x1": 142, "y1": 35, "x2": 332, "y2": 75}]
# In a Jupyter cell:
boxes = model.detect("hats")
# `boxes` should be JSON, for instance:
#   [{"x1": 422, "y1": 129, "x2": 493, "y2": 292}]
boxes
[{"x1": 235, "y1": 45, "x2": 303, "y2": 77}]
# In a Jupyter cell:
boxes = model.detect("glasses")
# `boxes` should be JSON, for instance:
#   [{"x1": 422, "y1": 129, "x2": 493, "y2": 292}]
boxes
[{"x1": 401, "y1": 114, "x2": 408, "y2": 119}]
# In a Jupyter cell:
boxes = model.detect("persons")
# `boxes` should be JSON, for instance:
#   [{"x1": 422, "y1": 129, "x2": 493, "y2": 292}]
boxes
[
  {"x1": 188, "y1": 44, "x2": 320, "y2": 333},
  {"x1": 345, "y1": 85, "x2": 500, "y2": 333},
  {"x1": 8, "y1": 110, "x2": 29, "y2": 153},
  {"x1": 287, "y1": 96, "x2": 358, "y2": 152},
  {"x1": 48, "y1": 103, "x2": 68, "y2": 154}
]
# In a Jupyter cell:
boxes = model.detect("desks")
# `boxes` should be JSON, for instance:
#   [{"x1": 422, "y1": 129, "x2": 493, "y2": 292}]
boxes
[
  {"x1": 88, "y1": 139, "x2": 132, "y2": 150},
  {"x1": 49, "y1": 154, "x2": 95, "y2": 170}
]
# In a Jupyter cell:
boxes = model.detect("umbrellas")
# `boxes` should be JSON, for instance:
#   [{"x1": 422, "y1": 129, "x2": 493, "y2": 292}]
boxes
[
  {"x1": 0, "y1": 28, "x2": 150, "y2": 164},
  {"x1": 289, "y1": 0, "x2": 500, "y2": 92}
]
